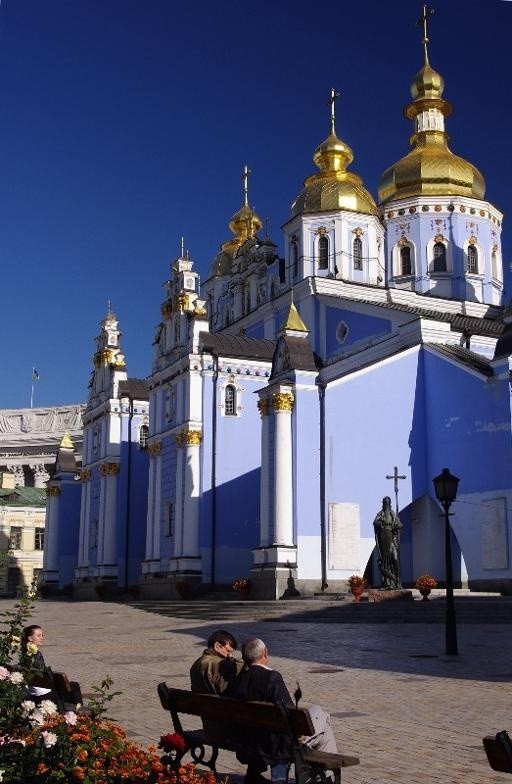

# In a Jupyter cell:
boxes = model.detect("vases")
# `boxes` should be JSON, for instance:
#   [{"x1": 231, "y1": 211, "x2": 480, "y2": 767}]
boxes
[
  {"x1": 239, "y1": 588, "x2": 253, "y2": 601},
  {"x1": 419, "y1": 586, "x2": 433, "y2": 602},
  {"x1": 351, "y1": 586, "x2": 364, "y2": 604}
]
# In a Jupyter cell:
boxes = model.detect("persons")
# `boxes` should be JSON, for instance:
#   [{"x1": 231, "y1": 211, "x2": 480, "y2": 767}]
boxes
[
  {"x1": 231, "y1": 638, "x2": 338, "y2": 784},
  {"x1": 20, "y1": 625, "x2": 83, "y2": 706},
  {"x1": 190, "y1": 630, "x2": 271, "y2": 784},
  {"x1": 373, "y1": 496, "x2": 404, "y2": 590}
]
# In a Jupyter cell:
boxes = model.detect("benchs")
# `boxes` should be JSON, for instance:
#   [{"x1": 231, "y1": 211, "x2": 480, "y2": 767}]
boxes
[
  {"x1": 7, "y1": 665, "x2": 85, "y2": 713},
  {"x1": 482, "y1": 731, "x2": 512, "y2": 775},
  {"x1": 157, "y1": 682, "x2": 361, "y2": 784}
]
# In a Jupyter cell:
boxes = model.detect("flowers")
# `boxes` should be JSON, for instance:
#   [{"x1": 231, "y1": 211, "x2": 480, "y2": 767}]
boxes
[
  {"x1": 232, "y1": 577, "x2": 252, "y2": 591},
  {"x1": 347, "y1": 573, "x2": 368, "y2": 587},
  {"x1": 414, "y1": 573, "x2": 439, "y2": 589}
]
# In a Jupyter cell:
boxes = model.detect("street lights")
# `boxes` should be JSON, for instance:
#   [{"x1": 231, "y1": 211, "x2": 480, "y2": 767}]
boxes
[{"x1": 433, "y1": 468, "x2": 462, "y2": 658}]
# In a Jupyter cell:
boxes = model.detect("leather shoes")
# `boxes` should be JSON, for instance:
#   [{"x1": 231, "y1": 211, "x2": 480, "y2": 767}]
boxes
[{"x1": 244, "y1": 775, "x2": 274, "y2": 784}]
